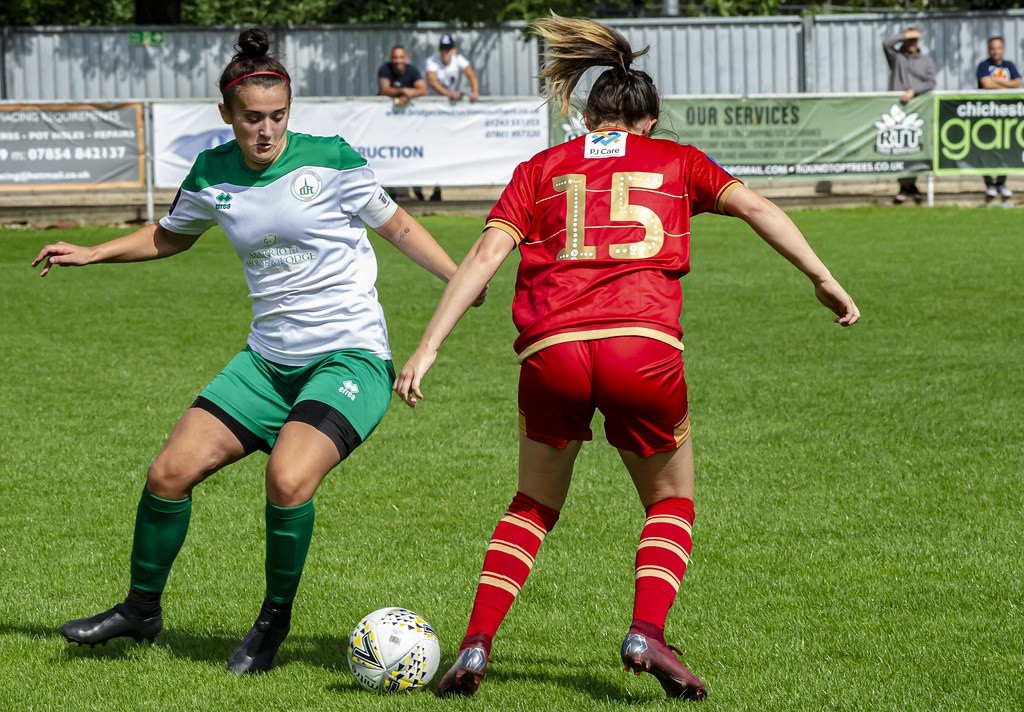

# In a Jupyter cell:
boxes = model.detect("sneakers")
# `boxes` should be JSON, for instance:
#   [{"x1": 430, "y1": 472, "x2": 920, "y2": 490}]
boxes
[
  {"x1": 61, "y1": 602, "x2": 164, "y2": 649},
  {"x1": 621, "y1": 632, "x2": 708, "y2": 701},
  {"x1": 438, "y1": 644, "x2": 489, "y2": 697},
  {"x1": 225, "y1": 605, "x2": 292, "y2": 677}
]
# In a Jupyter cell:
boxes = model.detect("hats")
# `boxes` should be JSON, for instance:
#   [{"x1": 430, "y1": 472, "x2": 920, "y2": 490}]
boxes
[{"x1": 440, "y1": 36, "x2": 454, "y2": 51}]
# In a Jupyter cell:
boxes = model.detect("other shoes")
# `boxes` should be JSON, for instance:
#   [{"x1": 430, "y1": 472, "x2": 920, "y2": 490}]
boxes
[
  {"x1": 987, "y1": 186, "x2": 998, "y2": 198},
  {"x1": 998, "y1": 184, "x2": 1013, "y2": 198},
  {"x1": 895, "y1": 192, "x2": 909, "y2": 201},
  {"x1": 431, "y1": 192, "x2": 442, "y2": 202}
]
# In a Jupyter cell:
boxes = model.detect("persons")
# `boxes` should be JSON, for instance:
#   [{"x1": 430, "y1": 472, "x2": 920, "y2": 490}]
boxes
[
  {"x1": 376, "y1": 45, "x2": 428, "y2": 200},
  {"x1": 391, "y1": 11, "x2": 862, "y2": 701},
  {"x1": 883, "y1": 29, "x2": 935, "y2": 204},
  {"x1": 33, "y1": 32, "x2": 489, "y2": 680},
  {"x1": 976, "y1": 36, "x2": 1022, "y2": 200},
  {"x1": 413, "y1": 34, "x2": 477, "y2": 200}
]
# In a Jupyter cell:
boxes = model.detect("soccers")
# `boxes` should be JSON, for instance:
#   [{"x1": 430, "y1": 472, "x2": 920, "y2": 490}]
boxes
[{"x1": 346, "y1": 605, "x2": 442, "y2": 694}]
